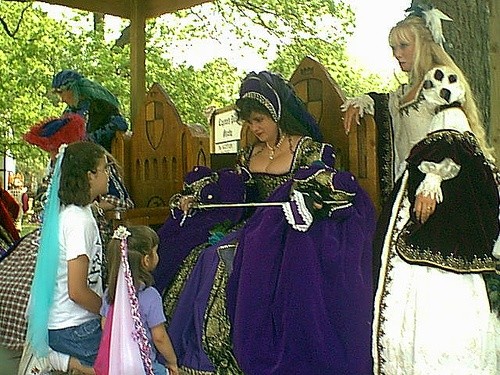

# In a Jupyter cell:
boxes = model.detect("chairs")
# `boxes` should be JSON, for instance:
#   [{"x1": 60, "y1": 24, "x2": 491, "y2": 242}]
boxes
[
  {"x1": 239, "y1": 56, "x2": 382, "y2": 214},
  {"x1": 102, "y1": 81, "x2": 209, "y2": 228}
]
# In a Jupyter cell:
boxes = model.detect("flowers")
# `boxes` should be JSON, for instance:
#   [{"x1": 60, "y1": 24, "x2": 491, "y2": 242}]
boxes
[
  {"x1": 54, "y1": 144, "x2": 68, "y2": 157},
  {"x1": 114, "y1": 226, "x2": 131, "y2": 240}
]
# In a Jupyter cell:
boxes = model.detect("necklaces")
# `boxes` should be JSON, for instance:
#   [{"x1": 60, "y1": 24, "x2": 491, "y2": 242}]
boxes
[{"x1": 264, "y1": 133, "x2": 286, "y2": 160}]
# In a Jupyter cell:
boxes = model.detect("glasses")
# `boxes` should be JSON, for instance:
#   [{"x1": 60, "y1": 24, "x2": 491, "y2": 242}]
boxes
[{"x1": 89, "y1": 166, "x2": 111, "y2": 176}]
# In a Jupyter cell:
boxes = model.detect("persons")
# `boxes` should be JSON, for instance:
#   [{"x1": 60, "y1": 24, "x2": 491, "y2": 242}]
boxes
[
  {"x1": 17, "y1": 141, "x2": 112, "y2": 375},
  {"x1": 0, "y1": 71, "x2": 135, "y2": 350},
  {"x1": 148, "y1": 69, "x2": 378, "y2": 375},
  {"x1": 341, "y1": 0, "x2": 500, "y2": 375},
  {"x1": 94, "y1": 223, "x2": 181, "y2": 375}
]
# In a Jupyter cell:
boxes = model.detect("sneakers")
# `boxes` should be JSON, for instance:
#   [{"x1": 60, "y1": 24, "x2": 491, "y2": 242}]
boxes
[{"x1": 17, "y1": 340, "x2": 52, "y2": 375}]
